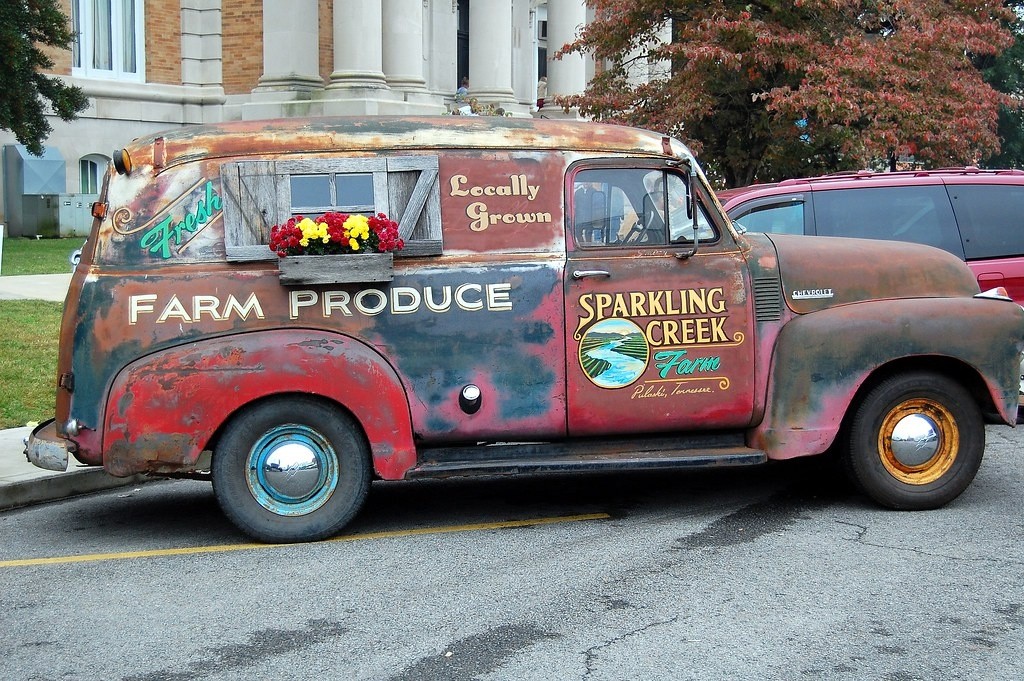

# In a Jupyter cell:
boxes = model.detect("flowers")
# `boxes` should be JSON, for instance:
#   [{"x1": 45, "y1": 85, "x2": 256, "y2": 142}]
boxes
[{"x1": 266, "y1": 212, "x2": 404, "y2": 256}]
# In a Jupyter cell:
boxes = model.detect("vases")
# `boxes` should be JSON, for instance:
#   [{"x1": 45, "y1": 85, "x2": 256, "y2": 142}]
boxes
[{"x1": 277, "y1": 252, "x2": 395, "y2": 285}]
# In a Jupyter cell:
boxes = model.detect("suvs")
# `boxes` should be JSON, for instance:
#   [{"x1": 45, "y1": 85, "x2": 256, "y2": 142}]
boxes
[
  {"x1": 22, "y1": 108, "x2": 1024, "y2": 540},
  {"x1": 662, "y1": 165, "x2": 1024, "y2": 312}
]
